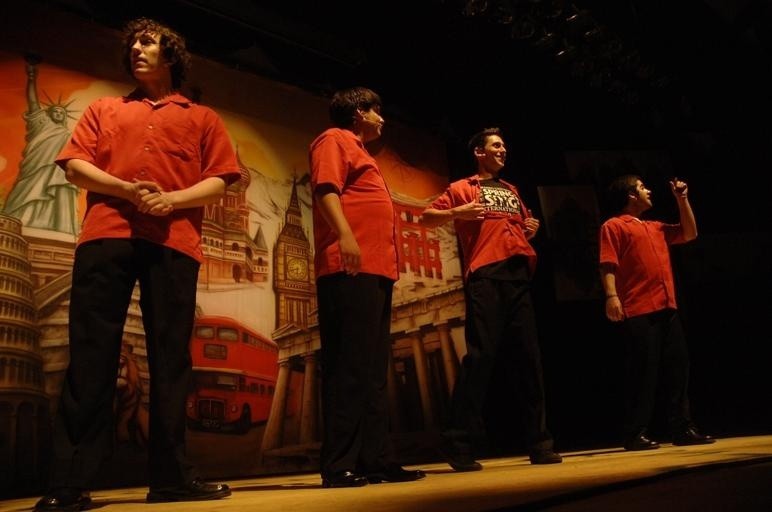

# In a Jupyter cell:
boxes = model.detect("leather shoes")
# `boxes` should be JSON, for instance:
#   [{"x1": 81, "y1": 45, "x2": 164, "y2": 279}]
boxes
[
  {"x1": 32, "y1": 488, "x2": 94, "y2": 512},
  {"x1": 316, "y1": 466, "x2": 426, "y2": 486},
  {"x1": 672, "y1": 424, "x2": 715, "y2": 446},
  {"x1": 514, "y1": 426, "x2": 563, "y2": 464},
  {"x1": 620, "y1": 430, "x2": 662, "y2": 450},
  {"x1": 145, "y1": 475, "x2": 231, "y2": 502},
  {"x1": 431, "y1": 432, "x2": 484, "y2": 471}
]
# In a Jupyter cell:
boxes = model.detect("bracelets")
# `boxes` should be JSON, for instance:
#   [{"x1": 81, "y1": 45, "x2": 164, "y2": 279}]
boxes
[{"x1": 607, "y1": 294, "x2": 618, "y2": 299}]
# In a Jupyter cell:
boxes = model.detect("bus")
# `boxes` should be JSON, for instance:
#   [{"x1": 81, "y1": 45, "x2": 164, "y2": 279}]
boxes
[{"x1": 185, "y1": 315, "x2": 301, "y2": 437}]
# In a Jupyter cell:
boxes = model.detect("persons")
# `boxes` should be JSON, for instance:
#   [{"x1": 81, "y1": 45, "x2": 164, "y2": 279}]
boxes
[
  {"x1": 598, "y1": 175, "x2": 716, "y2": 451},
  {"x1": 34, "y1": 20, "x2": 241, "y2": 510},
  {"x1": 307, "y1": 88, "x2": 426, "y2": 487},
  {"x1": 422, "y1": 127, "x2": 562, "y2": 470}
]
